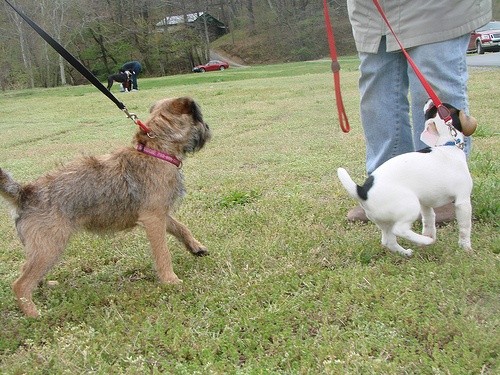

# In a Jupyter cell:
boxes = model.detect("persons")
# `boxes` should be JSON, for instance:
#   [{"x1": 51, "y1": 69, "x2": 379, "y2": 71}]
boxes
[
  {"x1": 120, "y1": 61, "x2": 142, "y2": 91},
  {"x1": 347, "y1": 0, "x2": 492, "y2": 223}
]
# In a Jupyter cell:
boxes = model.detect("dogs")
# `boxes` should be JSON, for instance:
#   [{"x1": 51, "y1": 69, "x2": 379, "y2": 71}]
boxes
[
  {"x1": 338, "y1": 98, "x2": 477, "y2": 257},
  {"x1": 107, "y1": 68, "x2": 135, "y2": 93},
  {"x1": 0, "y1": 97, "x2": 212, "y2": 317}
]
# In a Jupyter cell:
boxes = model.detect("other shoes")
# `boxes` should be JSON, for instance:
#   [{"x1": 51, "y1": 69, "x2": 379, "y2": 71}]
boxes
[
  {"x1": 431, "y1": 203, "x2": 456, "y2": 223},
  {"x1": 348, "y1": 205, "x2": 368, "y2": 221},
  {"x1": 132, "y1": 89, "x2": 137, "y2": 92}
]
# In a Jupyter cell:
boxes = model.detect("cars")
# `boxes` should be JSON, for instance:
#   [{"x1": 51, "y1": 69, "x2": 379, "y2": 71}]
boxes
[
  {"x1": 467, "y1": 21, "x2": 500, "y2": 55},
  {"x1": 191, "y1": 60, "x2": 229, "y2": 73}
]
